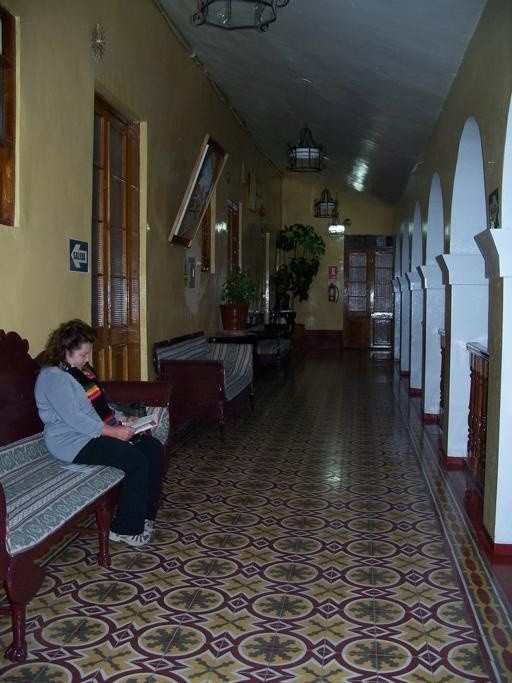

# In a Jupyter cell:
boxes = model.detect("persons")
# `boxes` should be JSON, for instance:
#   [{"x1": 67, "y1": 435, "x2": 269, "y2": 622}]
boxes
[{"x1": 34, "y1": 318, "x2": 166, "y2": 547}]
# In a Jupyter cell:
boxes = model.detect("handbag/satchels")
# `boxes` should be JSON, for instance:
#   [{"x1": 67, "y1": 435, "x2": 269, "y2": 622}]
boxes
[{"x1": 109, "y1": 398, "x2": 153, "y2": 437}]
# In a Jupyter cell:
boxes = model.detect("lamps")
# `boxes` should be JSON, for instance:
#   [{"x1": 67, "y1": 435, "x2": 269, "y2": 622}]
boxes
[
  {"x1": 313, "y1": 184, "x2": 346, "y2": 236},
  {"x1": 190, "y1": 0, "x2": 289, "y2": 32},
  {"x1": 285, "y1": 81, "x2": 326, "y2": 172}
]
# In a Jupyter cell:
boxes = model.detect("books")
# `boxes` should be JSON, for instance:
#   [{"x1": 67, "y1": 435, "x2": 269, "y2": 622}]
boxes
[{"x1": 121, "y1": 414, "x2": 159, "y2": 434}]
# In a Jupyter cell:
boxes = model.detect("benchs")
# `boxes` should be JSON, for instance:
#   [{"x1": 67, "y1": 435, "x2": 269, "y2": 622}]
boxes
[
  {"x1": 0, "y1": 330, "x2": 180, "y2": 665},
  {"x1": 155, "y1": 330, "x2": 256, "y2": 444}
]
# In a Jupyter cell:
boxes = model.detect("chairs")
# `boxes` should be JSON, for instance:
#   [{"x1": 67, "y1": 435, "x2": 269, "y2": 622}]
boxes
[{"x1": 249, "y1": 325, "x2": 294, "y2": 397}]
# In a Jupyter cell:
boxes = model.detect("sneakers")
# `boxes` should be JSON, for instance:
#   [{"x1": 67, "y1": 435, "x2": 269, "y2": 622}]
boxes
[{"x1": 107, "y1": 518, "x2": 155, "y2": 547}]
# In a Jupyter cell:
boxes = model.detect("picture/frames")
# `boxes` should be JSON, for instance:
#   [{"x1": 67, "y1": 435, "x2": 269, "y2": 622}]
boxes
[{"x1": 167, "y1": 131, "x2": 229, "y2": 248}]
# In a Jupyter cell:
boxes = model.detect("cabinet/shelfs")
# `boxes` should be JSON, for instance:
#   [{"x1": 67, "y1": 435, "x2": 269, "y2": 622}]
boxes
[{"x1": 342, "y1": 233, "x2": 395, "y2": 348}]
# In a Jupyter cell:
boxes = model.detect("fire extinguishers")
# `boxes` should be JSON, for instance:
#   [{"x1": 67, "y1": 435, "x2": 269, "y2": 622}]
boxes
[{"x1": 328, "y1": 282, "x2": 339, "y2": 304}]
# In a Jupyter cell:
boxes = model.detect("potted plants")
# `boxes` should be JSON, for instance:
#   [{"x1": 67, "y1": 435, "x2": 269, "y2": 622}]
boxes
[{"x1": 219, "y1": 268, "x2": 261, "y2": 330}]
